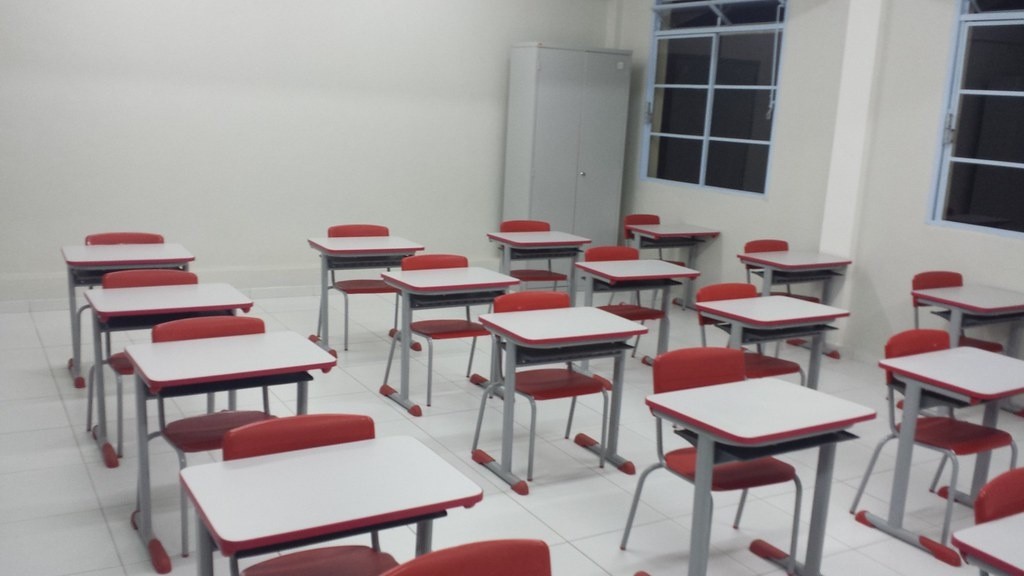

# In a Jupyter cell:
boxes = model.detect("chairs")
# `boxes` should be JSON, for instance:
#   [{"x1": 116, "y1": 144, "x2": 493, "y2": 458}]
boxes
[
  {"x1": 307, "y1": 212, "x2": 1024, "y2": 576},
  {"x1": 380, "y1": 538, "x2": 551, "y2": 576},
  {"x1": 84, "y1": 232, "x2": 164, "y2": 358},
  {"x1": 87, "y1": 270, "x2": 198, "y2": 458},
  {"x1": 152, "y1": 316, "x2": 276, "y2": 557},
  {"x1": 223, "y1": 413, "x2": 400, "y2": 576}
]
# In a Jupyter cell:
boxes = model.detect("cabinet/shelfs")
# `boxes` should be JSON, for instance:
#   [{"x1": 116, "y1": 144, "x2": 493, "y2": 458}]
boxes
[{"x1": 502, "y1": 41, "x2": 634, "y2": 293}]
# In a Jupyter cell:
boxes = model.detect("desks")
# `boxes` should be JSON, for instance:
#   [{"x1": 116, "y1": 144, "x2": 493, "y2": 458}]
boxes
[
  {"x1": 854, "y1": 346, "x2": 1024, "y2": 565},
  {"x1": 307, "y1": 236, "x2": 425, "y2": 357},
  {"x1": 645, "y1": 378, "x2": 876, "y2": 576},
  {"x1": 470, "y1": 304, "x2": 648, "y2": 496},
  {"x1": 736, "y1": 251, "x2": 852, "y2": 360},
  {"x1": 627, "y1": 222, "x2": 720, "y2": 311},
  {"x1": 951, "y1": 512, "x2": 1024, "y2": 576},
  {"x1": 486, "y1": 231, "x2": 591, "y2": 306},
  {"x1": 178, "y1": 435, "x2": 483, "y2": 576},
  {"x1": 86, "y1": 281, "x2": 253, "y2": 469},
  {"x1": 910, "y1": 284, "x2": 1024, "y2": 349},
  {"x1": 695, "y1": 294, "x2": 850, "y2": 390},
  {"x1": 574, "y1": 259, "x2": 700, "y2": 367},
  {"x1": 60, "y1": 244, "x2": 194, "y2": 390},
  {"x1": 123, "y1": 330, "x2": 336, "y2": 574},
  {"x1": 380, "y1": 268, "x2": 520, "y2": 418}
]
